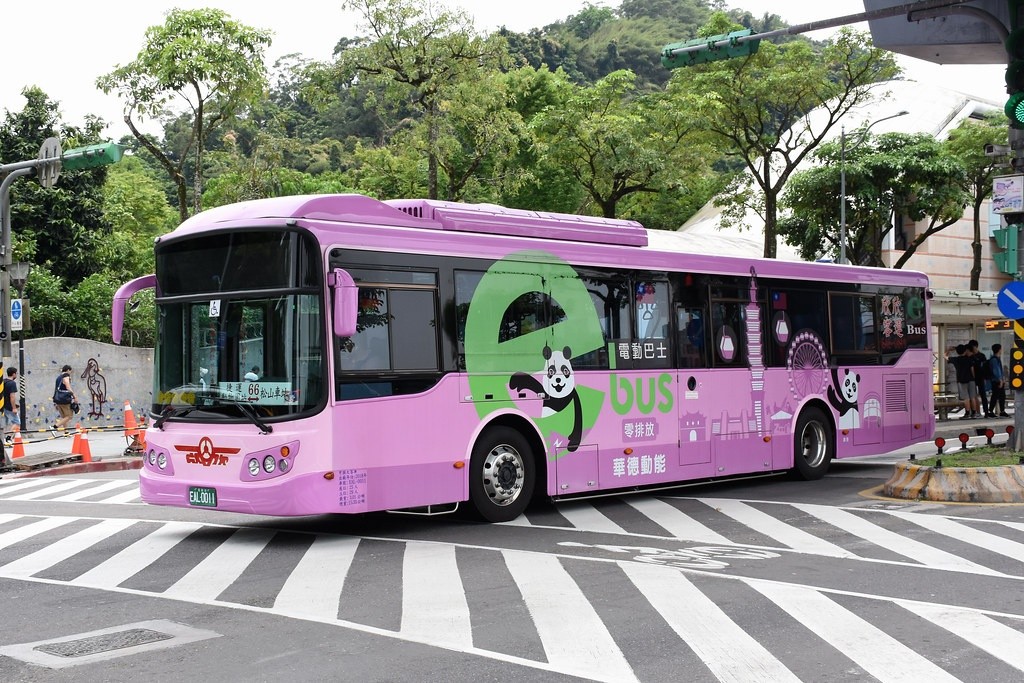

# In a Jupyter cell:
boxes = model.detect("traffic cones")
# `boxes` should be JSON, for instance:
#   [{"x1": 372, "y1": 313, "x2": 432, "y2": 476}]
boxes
[
  {"x1": 11, "y1": 426, "x2": 25, "y2": 459},
  {"x1": 68, "y1": 422, "x2": 81, "y2": 460},
  {"x1": 75, "y1": 427, "x2": 94, "y2": 463},
  {"x1": 127, "y1": 415, "x2": 146, "y2": 453},
  {"x1": 123, "y1": 400, "x2": 141, "y2": 436}
]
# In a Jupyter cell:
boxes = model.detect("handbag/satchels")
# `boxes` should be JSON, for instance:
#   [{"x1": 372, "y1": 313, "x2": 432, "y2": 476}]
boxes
[{"x1": 53, "y1": 390, "x2": 73, "y2": 404}]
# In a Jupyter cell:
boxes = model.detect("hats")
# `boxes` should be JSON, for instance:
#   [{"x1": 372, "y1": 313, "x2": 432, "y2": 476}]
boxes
[{"x1": 71, "y1": 403, "x2": 79, "y2": 415}]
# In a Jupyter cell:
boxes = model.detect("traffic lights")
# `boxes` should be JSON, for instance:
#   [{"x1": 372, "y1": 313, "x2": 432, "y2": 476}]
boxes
[{"x1": 1003, "y1": 29, "x2": 1024, "y2": 127}]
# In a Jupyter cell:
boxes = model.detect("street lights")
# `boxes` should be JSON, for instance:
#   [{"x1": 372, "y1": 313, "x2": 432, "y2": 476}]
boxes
[{"x1": 840, "y1": 110, "x2": 911, "y2": 264}]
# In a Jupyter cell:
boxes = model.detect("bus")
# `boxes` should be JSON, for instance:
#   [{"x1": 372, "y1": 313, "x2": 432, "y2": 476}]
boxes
[{"x1": 112, "y1": 191, "x2": 936, "y2": 524}]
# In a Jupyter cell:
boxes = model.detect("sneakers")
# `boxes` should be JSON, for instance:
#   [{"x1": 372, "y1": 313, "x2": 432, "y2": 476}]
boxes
[
  {"x1": 4, "y1": 437, "x2": 14, "y2": 448},
  {"x1": 959, "y1": 412, "x2": 1012, "y2": 420},
  {"x1": 50, "y1": 426, "x2": 58, "y2": 437}
]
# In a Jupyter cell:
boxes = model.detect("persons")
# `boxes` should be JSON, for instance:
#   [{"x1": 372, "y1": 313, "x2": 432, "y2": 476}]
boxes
[
  {"x1": 200, "y1": 366, "x2": 208, "y2": 391},
  {"x1": 4, "y1": 367, "x2": 21, "y2": 448},
  {"x1": 244, "y1": 365, "x2": 260, "y2": 382},
  {"x1": 50, "y1": 365, "x2": 79, "y2": 437},
  {"x1": 342, "y1": 329, "x2": 382, "y2": 369},
  {"x1": 943, "y1": 339, "x2": 1009, "y2": 420}
]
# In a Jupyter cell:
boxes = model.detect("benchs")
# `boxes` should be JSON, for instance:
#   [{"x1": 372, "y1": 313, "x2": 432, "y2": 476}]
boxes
[{"x1": 935, "y1": 397, "x2": 1000, "y2": 419}]
